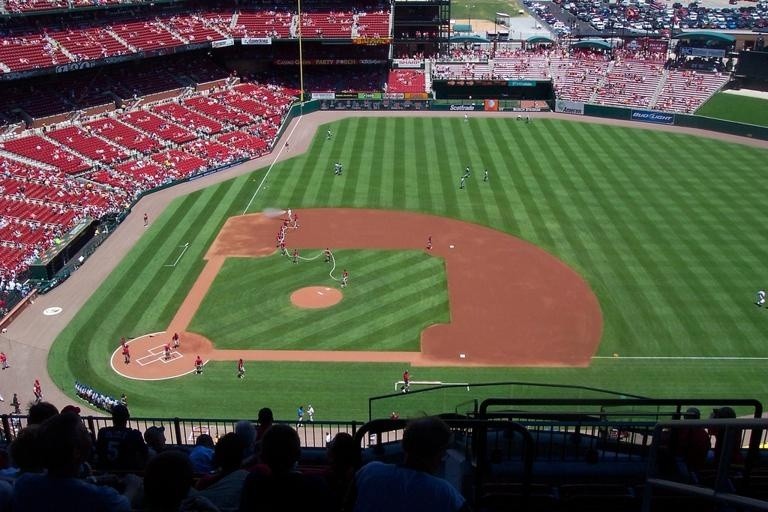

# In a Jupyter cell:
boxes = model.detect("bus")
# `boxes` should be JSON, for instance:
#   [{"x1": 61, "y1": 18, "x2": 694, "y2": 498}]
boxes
[{"x1": 486, "y1": 30, "x2": 509, "y2": 42}]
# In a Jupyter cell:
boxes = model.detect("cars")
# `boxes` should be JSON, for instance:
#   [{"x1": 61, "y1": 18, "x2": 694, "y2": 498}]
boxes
[{"x1": 523, "y1": 0, "x2": 768, "y2": 38}]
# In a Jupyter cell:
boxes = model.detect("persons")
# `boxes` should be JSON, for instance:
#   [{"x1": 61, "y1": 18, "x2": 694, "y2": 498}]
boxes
[
  {"x1": 757, "y1": 290, "x2": 766, "y2": 304},
  {"x1": 0, "y1": 0, "x2": 742, "y2": 512}
]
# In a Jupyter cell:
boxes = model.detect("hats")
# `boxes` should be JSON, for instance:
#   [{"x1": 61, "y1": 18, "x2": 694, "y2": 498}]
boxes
[
  {"x1": 144, "y1": 426, "x2": 164, "y2": 442},
  {"x1": 60, "y1": 405, "x2": 80, "y2": 415},
  {"x1": 258, "y1": 408, "x2": 273, "y2": 423}
]
